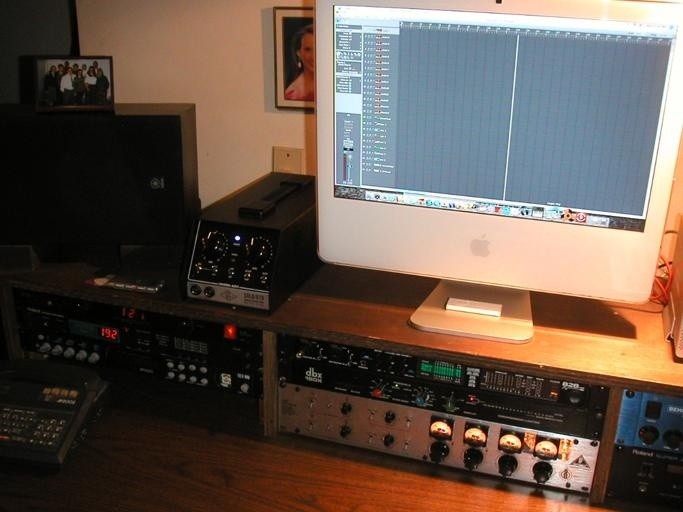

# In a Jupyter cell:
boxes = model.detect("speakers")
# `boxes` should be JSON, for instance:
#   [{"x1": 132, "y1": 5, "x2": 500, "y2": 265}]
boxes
[{"x1": 0, "y1": 102, "x2": 200, "y2": 271}]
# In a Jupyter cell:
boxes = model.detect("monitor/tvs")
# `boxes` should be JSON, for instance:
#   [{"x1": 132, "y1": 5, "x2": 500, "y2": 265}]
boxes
[{"x1": 316, "y1": 0, "x2": 683, "y2": 345}]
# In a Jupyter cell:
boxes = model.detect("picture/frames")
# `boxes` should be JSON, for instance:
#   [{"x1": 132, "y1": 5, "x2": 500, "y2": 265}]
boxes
[
  {"x1": 273, "y1": 5, "x2": 316, "y2": 112},
  {"x1": 32, "y1": 54, "x2": 116, "y2": 112}
]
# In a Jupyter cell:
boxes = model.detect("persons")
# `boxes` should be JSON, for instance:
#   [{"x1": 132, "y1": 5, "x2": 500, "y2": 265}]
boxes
[
  {"x1": 285, "y1": 24, "x2": 315, "y2": 101},
  {"x1": 39, "y1": 57, "x2": 109, "y2": 107}
]
